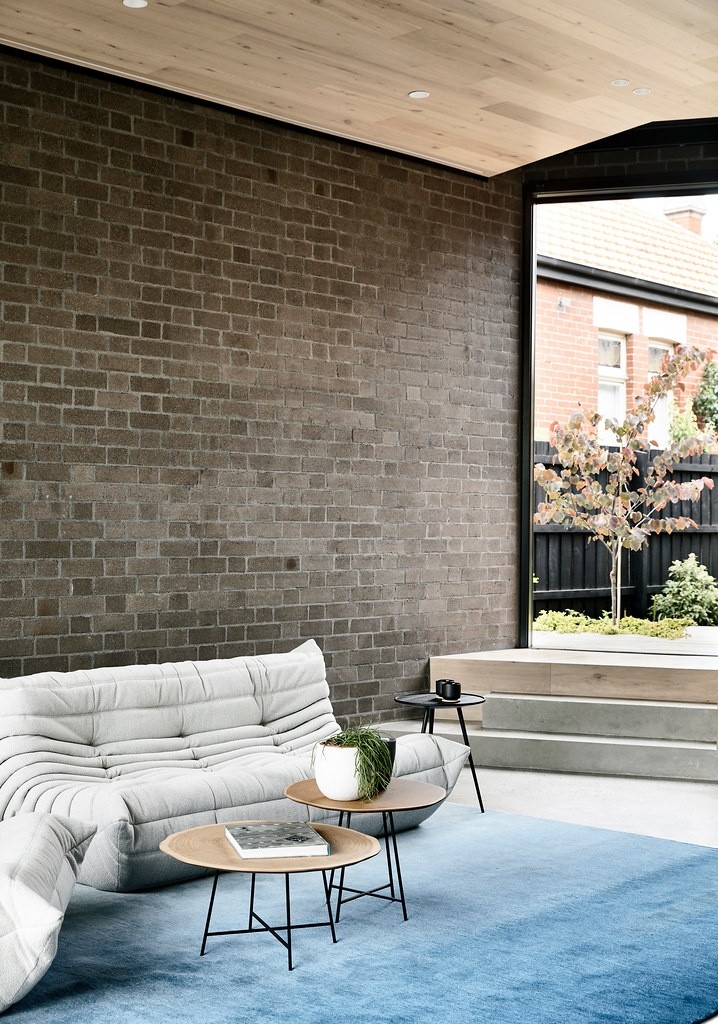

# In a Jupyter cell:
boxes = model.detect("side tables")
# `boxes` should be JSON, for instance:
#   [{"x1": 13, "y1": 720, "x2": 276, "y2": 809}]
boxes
[{"x1": 395, "y1": 694, "x2": 487, "y2": 812}]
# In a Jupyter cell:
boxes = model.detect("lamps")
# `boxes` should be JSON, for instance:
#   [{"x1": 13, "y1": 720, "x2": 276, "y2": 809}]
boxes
[{"x1": 558, "y1": 297, "x2": 573, "y2": 308}]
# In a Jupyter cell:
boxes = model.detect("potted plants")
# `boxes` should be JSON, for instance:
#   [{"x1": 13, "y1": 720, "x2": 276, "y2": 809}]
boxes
[{"x1": 313, "y1": 727, "x2": 395, "y2": 802}]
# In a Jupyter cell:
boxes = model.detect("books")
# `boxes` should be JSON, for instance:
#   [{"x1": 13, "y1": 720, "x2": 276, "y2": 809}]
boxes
[{"x1": 223, "y1": 820, "x2": 331, "y2": 860}]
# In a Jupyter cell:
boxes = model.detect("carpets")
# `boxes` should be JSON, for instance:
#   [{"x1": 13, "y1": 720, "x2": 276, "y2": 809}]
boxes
[{"x1": 0, "y1": 806, "x2": 718, "y2": 1024}]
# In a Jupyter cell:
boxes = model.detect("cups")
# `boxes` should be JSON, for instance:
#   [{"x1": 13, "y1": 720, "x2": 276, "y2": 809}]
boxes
[{"x1": 435, "y1": 678, "x2": 462, "y2": 702}]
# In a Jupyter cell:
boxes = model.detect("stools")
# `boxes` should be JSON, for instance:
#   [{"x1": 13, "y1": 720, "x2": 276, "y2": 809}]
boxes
[{"x1": 0, "y1": 811, "x2": 97, "y2": 1015}]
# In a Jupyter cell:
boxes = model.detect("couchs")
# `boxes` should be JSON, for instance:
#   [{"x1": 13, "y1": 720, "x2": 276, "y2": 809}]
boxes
[{"x1": 0, "y1": 637, "x2": 469, "y2": 892}]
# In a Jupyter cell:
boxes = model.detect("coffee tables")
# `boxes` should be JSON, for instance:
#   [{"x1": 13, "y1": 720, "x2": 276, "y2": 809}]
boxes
[
  {"x1": 160, "y1": 820, "x2": 380, "y2": 972},
  {"x1": 284, "y1": 774, "x2": 446, "y2": 922}
]
